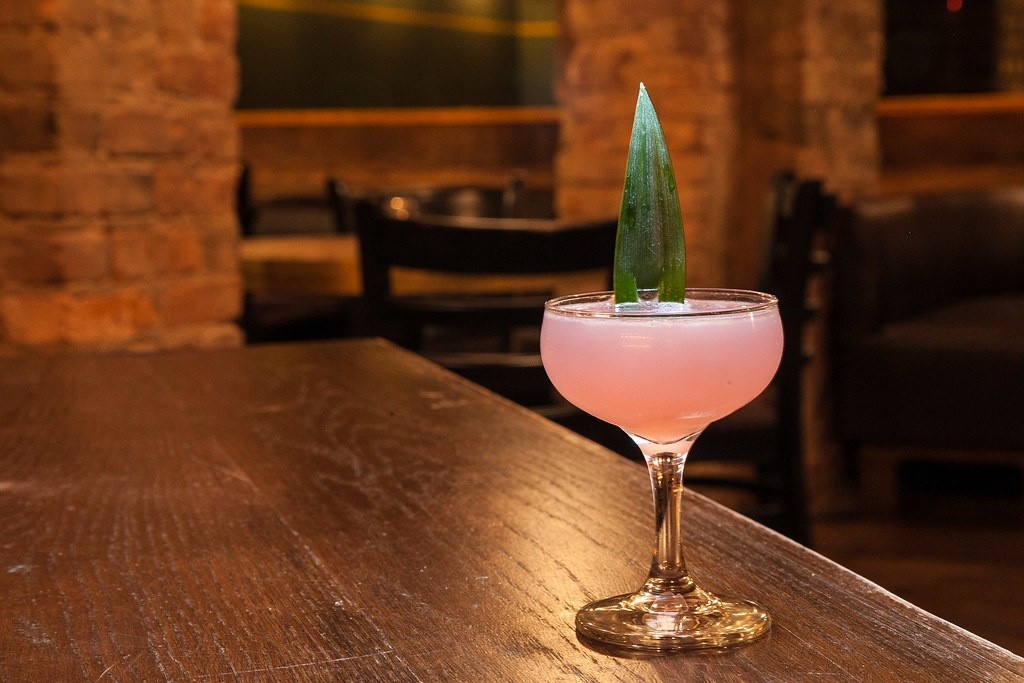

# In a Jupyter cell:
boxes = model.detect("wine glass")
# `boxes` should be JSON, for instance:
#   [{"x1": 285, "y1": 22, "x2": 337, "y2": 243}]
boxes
[{"x1": 537, "y1": 285, "x2": 783, "y2": 650}]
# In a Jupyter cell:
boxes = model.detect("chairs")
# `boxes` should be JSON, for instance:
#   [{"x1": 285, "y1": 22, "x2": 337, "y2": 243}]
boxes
[{"x1": 352, "y1": 206, "x2": 630, "y2": 450}]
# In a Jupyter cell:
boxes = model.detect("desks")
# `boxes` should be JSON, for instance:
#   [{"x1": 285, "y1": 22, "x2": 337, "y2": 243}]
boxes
[
  {"x1": 232, "y1": 232, "x2": 613, "y2": 314},
  {"x1": 0, "y1": 338, "x2": 1024, "y2": 683}
]
